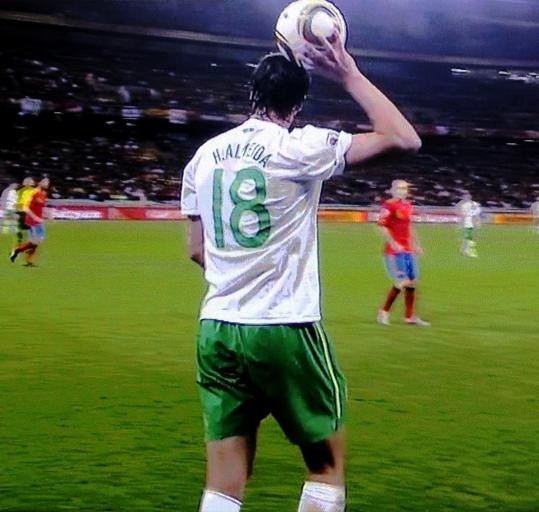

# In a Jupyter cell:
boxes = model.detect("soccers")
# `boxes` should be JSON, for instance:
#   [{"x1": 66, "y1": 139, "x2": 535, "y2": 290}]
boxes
[{"x1": 274, "y1": 1, "x2": 347, "y2": 72}]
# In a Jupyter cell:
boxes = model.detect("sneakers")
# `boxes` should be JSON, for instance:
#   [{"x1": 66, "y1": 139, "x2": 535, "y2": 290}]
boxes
[
  {"x1": 9, "y1": 249, "x2": 18, "y2": 263},
  {"x1": 376, "y1": 307, "x2": 392, "y2": 327},
  {"x1": 404, "y1": 315, "x2": 430, "y2": 327},
  {"x1": 22, "y1": 262, "x2": 40, "y2": 269}
]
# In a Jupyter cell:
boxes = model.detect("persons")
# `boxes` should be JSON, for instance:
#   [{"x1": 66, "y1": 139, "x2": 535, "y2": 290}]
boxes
[
  {"x1": 179, "y1": 30, "x2": 423, "y2": 512},
  {"x1": 0, "y1": 173, "x2": 53, "y2": 267},
  {"x1": 454, "y1": 190, "x2": 485, "y2": 261},
  {"x1": 376, "y1": 180, "x2": 432, "y2": 327}
]
